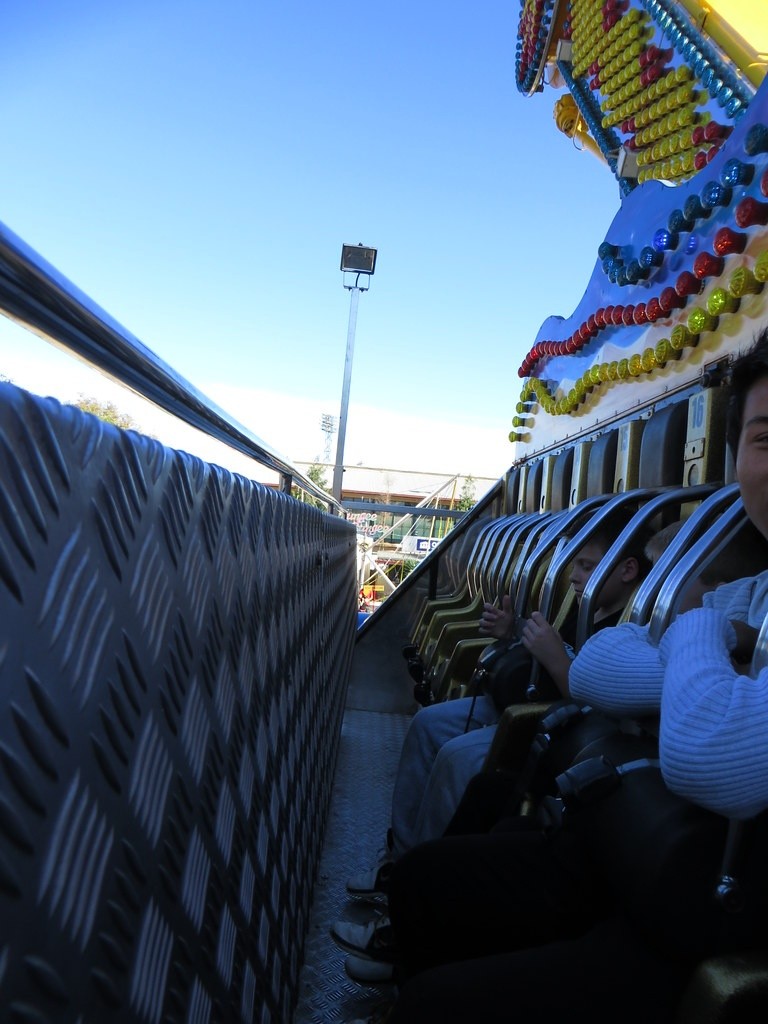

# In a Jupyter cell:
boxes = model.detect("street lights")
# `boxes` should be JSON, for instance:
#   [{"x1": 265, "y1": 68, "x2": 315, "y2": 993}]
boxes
[{"x1": 331, "y1": 242, "x2": 380, "y2": 501}]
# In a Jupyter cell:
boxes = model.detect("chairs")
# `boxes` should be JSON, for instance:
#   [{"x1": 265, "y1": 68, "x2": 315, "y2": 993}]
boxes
[{"x1": 406, "y1": 482, "x2": 768, "y2": 912}]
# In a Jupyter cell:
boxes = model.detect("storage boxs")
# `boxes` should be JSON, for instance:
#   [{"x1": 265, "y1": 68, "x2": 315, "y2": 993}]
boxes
[{"x1": 403, "y1": 535, "x2": 442, "y2": 556}]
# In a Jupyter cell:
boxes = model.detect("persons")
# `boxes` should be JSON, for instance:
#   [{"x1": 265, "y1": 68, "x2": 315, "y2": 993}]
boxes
[
  {"x1": 331, "y1": 513, "x2": 767, "y2": 985},
  {"x1": 385, "y1": 328, "x2": 768, "y2": 1023},
  {"x1": 346, "y1": 509, "x2": 647, "y2": 914}
]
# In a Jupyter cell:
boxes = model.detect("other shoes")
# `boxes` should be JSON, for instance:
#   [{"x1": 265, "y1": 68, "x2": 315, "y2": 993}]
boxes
[
  {"x1": 345, "y1": 851, "x2": 399, "y2": 897},
  {"x1": 344, "y1": 955, "x2": 396, "y2": 984},
  {"x1": 329, "y1": 912, "x2": 402, "y2": 962}
]
